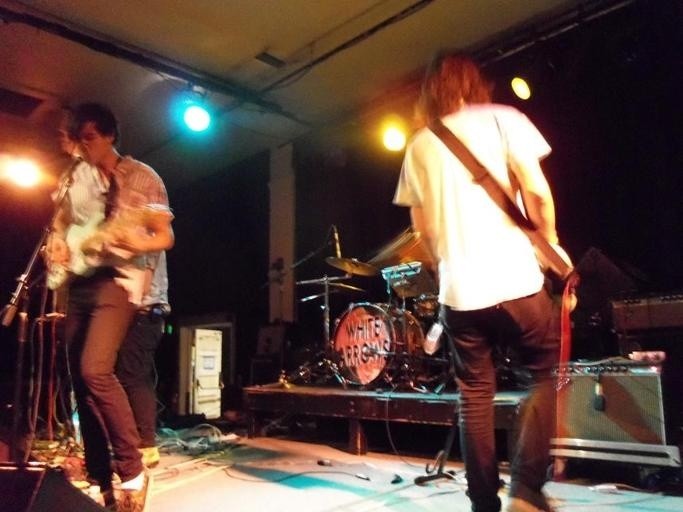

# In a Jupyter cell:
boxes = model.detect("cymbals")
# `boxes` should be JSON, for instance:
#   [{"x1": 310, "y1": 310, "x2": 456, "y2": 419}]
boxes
[{"x1": 326, "y1": 256, "x2": 380, "y2": 276}]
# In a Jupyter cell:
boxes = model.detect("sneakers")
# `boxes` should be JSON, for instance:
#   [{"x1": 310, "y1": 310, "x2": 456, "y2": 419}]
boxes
[
  {"x1": 504, "y1": 497, "x2": 544, "y2": 512},
  {"x1": 138, "y1": 446, "x2": 160, "y2": 468},
  {"x1": 114, "y1": 465, "x2": 151, "y2": 512}
]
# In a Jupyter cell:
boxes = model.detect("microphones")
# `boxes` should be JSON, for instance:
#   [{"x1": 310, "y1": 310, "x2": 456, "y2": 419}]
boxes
[
  {"x1": 334, "y1": 231, "x2": 342, "y2": 259},
  {"x1": 62, "y1": 151, "x2": 85, "y2": 179},
  {"x1": 594, "y1": 382, "x2": 607, "y2": 412}
]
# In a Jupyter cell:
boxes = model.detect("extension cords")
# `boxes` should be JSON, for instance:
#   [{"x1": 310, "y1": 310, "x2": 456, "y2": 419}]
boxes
[{"x1": 208, "y1": 433, "x2": 239, "y2": 444}]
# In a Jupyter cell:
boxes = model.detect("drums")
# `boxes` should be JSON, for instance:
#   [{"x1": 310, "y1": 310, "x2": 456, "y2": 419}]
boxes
[
  {"x1": 412, "y1": 297, "x2": 439, "y2": 317},
  {"x1": 330, "y1": 303, "x2": 424, "y2": 387}
]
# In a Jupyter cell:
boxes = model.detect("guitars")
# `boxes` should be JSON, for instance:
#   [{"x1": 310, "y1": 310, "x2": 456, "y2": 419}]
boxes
[{"x1": 65, "y1": 207, "x2": 173, "y2": 278}]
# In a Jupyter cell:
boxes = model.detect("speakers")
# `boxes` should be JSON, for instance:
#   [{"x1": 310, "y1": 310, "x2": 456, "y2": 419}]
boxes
[{"x1": 555, "y1": 367, "x2": 668, "y2": 446}]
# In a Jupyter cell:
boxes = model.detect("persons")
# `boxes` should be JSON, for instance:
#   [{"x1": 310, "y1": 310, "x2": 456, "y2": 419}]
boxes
[
  {"x1": 42, "y1": 100, "x2": 176, "y2": 511},
  {"x1": 114, "y1": 250, "x2": 172, "y2": 468},
  {"x1": 393, "y1": 45, "x2": 578, "y2": 512}
]
